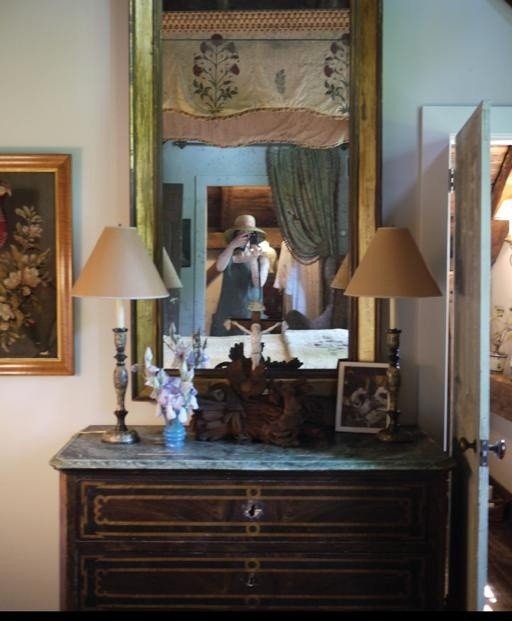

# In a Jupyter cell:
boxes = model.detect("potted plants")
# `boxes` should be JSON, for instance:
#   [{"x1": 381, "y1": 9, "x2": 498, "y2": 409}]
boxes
[{"x1": 489, "y1": 305, "x2": 512, "y2": 373}]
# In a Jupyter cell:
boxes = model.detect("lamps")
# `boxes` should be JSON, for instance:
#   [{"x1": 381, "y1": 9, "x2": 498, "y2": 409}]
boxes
[
  {"x1": 330, "y1": 253, "x2": 350, "y2": 289},
  {"x1": 163, "y1": 246, "x2": 184, "y2": 289},
  {"x1": 69, "y1": 224, "x2": 170, "y2": 444},
  {"x1": 343, "y1": 227, "x2": 444, "y2": 450}
]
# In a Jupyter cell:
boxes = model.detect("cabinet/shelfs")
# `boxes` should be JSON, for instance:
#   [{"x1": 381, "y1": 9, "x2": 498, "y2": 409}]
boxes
[{"x1": 61, "y1": 469, "x2": 456, "y2": 611}]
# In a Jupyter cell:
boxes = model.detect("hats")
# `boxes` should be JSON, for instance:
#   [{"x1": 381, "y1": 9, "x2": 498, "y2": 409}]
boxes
[{"x1": 222, "y1": 213, "x2": 268, "y2": 246}]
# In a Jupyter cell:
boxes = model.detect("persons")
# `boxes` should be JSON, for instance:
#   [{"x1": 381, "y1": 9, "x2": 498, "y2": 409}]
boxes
[{"x1": 210, "y1": 215, "x2": 277, "y2": 336}]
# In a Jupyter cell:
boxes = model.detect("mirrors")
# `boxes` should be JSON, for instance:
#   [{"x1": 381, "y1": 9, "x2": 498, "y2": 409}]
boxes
[{"x1": 131, "y1": 1, "x2": 382, "y2": 402}]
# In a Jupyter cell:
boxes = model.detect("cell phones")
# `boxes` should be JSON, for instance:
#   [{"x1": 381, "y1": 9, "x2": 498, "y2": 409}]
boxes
[
  {"x1": 250, "y1": 233, "x2": 258, "y2": 248},
  {"x1": 239, "y1": 235, "x2": 249, "y2": 251}
]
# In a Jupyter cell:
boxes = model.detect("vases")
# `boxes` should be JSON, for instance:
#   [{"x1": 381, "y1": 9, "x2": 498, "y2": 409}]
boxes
[{"x1": 161, "y1": 406, "x2": 188, "y2": 443}]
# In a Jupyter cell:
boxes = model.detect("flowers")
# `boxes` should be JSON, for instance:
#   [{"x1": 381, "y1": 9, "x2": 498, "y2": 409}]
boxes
[
  {"x1": 323, "y1": 33, "x2": 351, "y2": 112},
  {"x1": 192, "y1": 33, "x2": 240, "y2": 114},
  {"x1": 131, "y1": 347, "x2": 199, "y2": 426},
  {"x1": 162, "y1": 323, "x2": 207, "y2": 368}
]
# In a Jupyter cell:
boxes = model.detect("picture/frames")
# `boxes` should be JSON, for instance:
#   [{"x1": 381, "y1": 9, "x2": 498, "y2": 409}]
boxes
[
  {"x1": 0, "y1": 152, "x2": 74, "y2": 376},
  {"x1": 334, "y1": 358, "x2": 399, "y2": 438}
]
